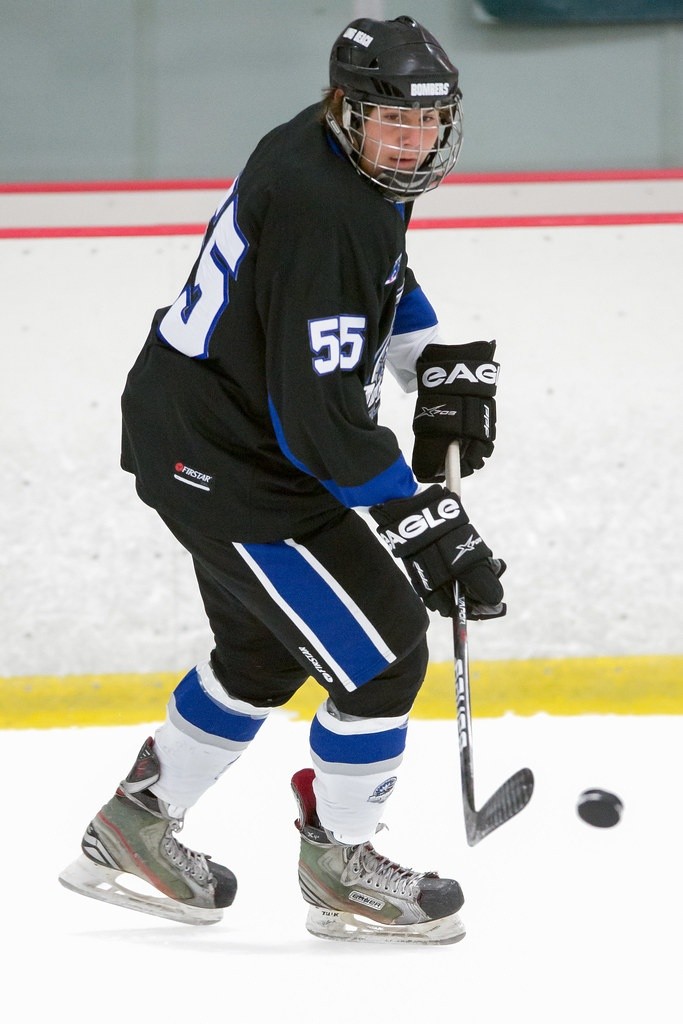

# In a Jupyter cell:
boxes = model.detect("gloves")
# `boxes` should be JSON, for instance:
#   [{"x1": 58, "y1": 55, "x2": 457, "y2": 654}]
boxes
[
  {"x1": 412, "y1": 340, "x2": 500, "y2": 484},
  {"x1": 368, "y1": 482, "x2": 507, "y2": 621}
]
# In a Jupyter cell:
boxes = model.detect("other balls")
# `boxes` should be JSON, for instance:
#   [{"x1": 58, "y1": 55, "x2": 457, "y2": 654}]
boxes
[{"x1": 578, "y1": 788, "x2": 624, "y2": 827}]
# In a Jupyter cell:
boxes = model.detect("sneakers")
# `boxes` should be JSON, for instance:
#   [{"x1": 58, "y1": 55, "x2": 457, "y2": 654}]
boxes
[
  {"x1": 56, "y1": 734, "x2": 237, "y2": 924},
  {"x1": 291, "y1": 767, "x2": 467, "y2": 945}
]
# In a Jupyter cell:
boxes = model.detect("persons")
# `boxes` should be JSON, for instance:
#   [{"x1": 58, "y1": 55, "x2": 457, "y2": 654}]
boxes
[{"x1": 61, "y1": 16, "x2": 506, "y2": 942}]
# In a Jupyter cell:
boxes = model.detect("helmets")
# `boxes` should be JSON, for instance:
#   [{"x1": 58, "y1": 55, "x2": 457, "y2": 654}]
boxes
[{"x1": 323, "y1": 16, "x2": 464, "y2": 205}]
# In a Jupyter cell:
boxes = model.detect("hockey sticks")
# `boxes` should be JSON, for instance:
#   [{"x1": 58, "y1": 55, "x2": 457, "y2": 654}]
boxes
[{"x1": 441, "y1": 441, "x2": 537, "y2": 845}]
